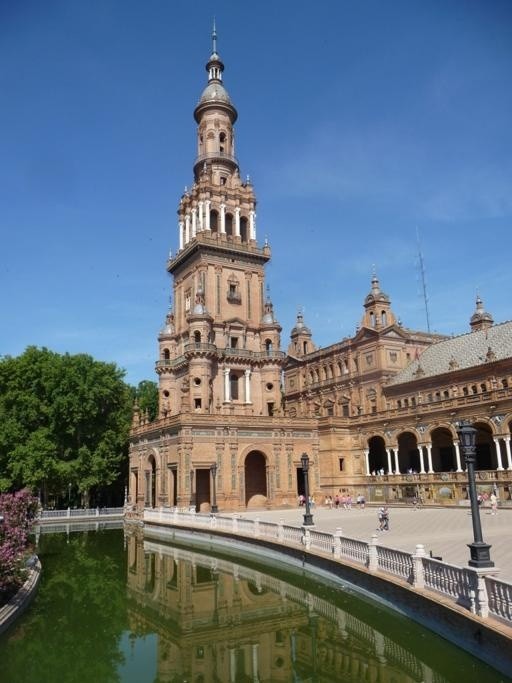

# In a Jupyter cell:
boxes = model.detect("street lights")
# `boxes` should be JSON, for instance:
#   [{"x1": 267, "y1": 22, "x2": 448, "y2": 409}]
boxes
[
  {"x1": 210, "y1": 462, "x2": 220, "y2": 513},
  {"x1": 300, "y1": 450, "x2": 316, "y2": 526},
  {"x1": 454, "y1": 416, "x2": 497, "y2": 566}
]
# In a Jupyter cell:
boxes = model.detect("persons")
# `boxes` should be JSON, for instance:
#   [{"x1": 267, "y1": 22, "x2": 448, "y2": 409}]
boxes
[{"x1": 298, "y1": 467, "x2": 501, "y2": 531}]
